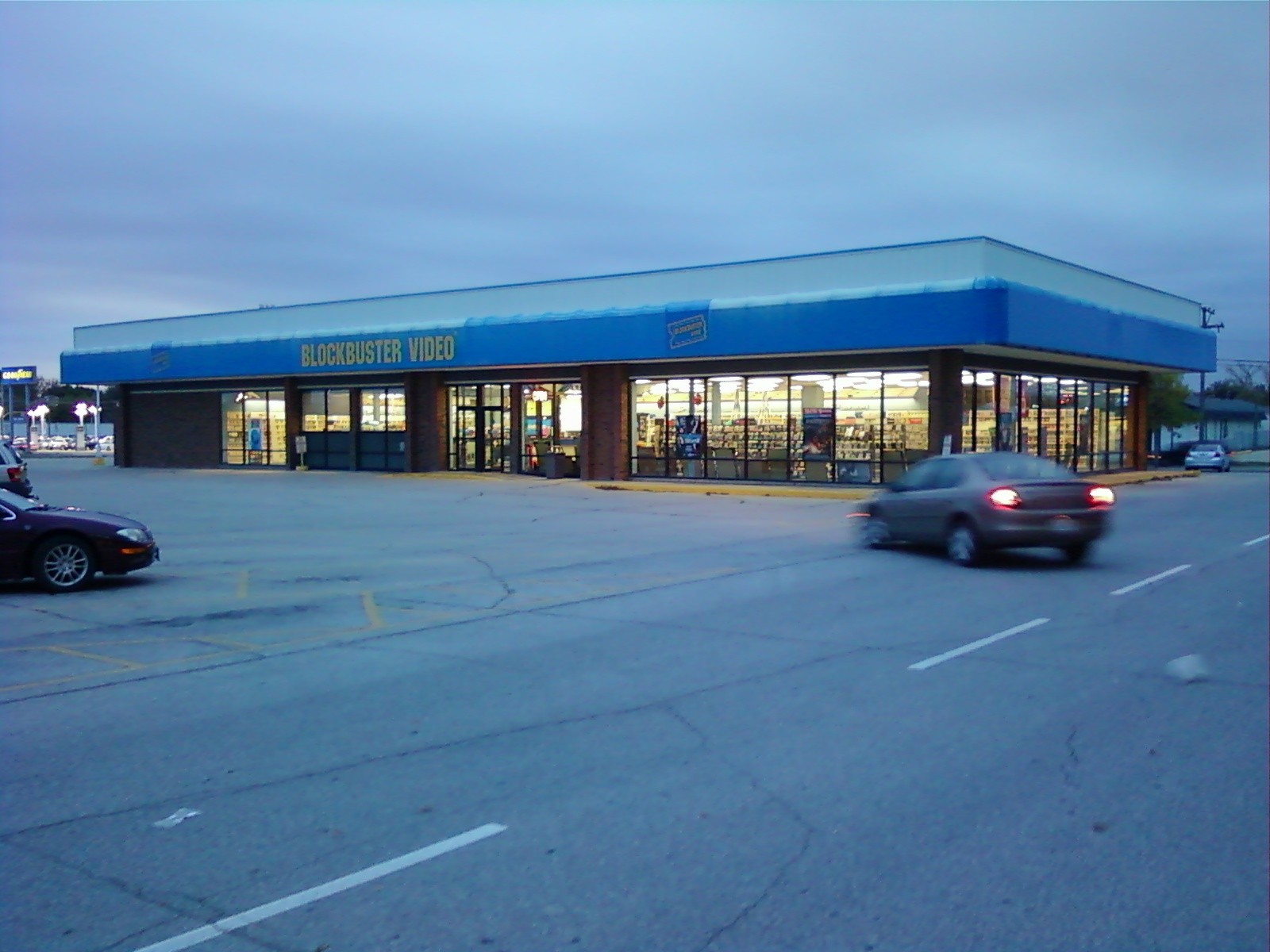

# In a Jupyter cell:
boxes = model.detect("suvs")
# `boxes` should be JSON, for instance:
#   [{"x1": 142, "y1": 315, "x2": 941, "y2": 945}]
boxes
[{"x1": 0, "y1": 439, "x2": 34, "y2": 499}]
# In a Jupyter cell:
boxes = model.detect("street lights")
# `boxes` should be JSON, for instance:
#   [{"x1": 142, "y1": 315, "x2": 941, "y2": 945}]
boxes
[
  {"x1": 88, "y1": 405, "x2": 101, "y2": 437},
  {"x1": 28, "y1": 409, "x2": 40, "y2": 443},
  {"x1": 34, "y1": 405, "x2": 50, "y2": 436},
  {"x1": 75, "y1": 402, "x2": 88, "y2": 451}
]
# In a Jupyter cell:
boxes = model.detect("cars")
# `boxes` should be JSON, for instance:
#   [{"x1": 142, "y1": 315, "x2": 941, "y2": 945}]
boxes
[
  {"x1": 0, "y1": 488, "x2": 160, "y2": 593},
  {"x1": 1185, "y1": 444, "x2": 1232, "y2": 473},
  {"x1": 858, "y1": 450, "x2": 1116, "y2": 567},
  {"x1": 0, "y1": 431, "x2": 115, "y2": 452}
]
